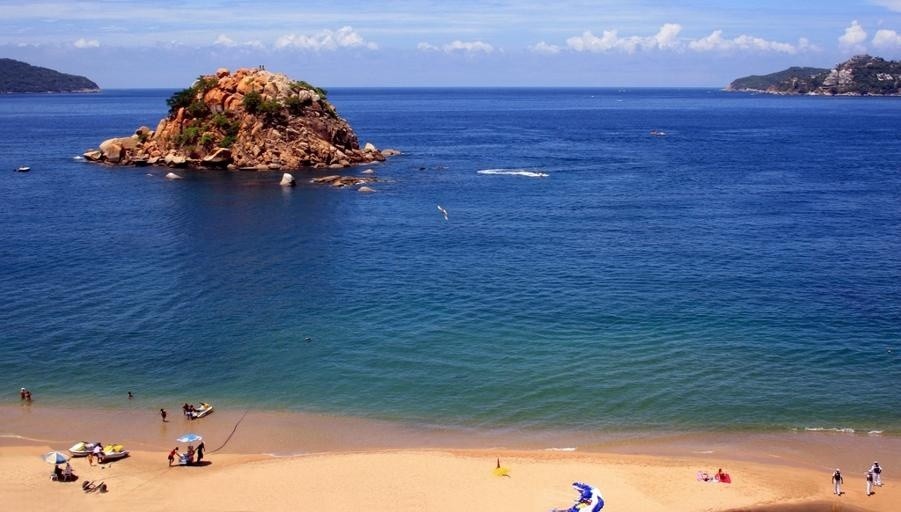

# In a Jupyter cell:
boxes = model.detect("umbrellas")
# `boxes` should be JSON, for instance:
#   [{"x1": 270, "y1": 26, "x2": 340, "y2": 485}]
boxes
[
  {"x1": 46, "y1": 451, "x2": 71, "y2": 464},
  {"x1": 176, "y1": 434, "x2": 203, "y2": 443}
]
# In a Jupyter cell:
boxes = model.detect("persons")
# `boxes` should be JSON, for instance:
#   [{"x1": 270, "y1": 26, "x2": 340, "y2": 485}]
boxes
[
  {"x1": 88, "y1": 443, "x2": 103, "y2": 465},
  {"x1": 55, "y1": 463, "x2": 72, "y2": 481},
  {"x1": 128, "y1": 392, "x2": 134, "y2": 399},
  {"x1": 832, "y1": 469, "x2": 844, "y2": 496},
  {"x1": 168, "y1": 442, "x2": 205, "y2": 467},
  {"x1": 866, "y1": 461, "x2": 883, "y2": 496},
  {"x1": 161, "y1": 408, "x2": 167, "y2": 422},
  {"x1": 21, "y1": 388, "x2": 32, "y2": 401},
  {"x1": 183, "y1": 403, "x2": 196, "y2": 420}
]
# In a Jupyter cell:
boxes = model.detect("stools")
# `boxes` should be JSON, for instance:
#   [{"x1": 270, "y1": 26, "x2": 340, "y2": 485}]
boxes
[{"x1": 50, "y1": 472, "x2": 73, "y2": 480}]
